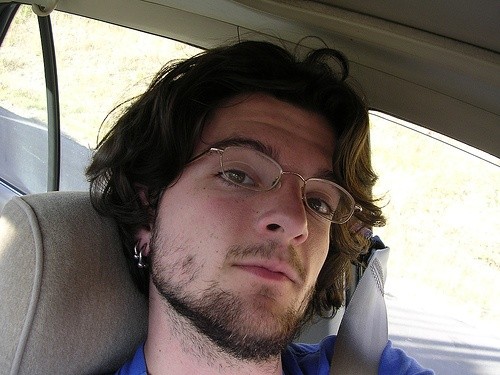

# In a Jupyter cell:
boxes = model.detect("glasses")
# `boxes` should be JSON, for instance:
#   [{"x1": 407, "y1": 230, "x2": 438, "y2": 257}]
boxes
[{"x1": 181, "y1": 146, "x2": 363, "y2": 225}]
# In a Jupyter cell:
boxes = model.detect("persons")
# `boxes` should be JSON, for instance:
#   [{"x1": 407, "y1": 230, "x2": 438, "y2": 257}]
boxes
[{"x1": 84, "y1": 35, "x2": 438, "y2": 375}]
